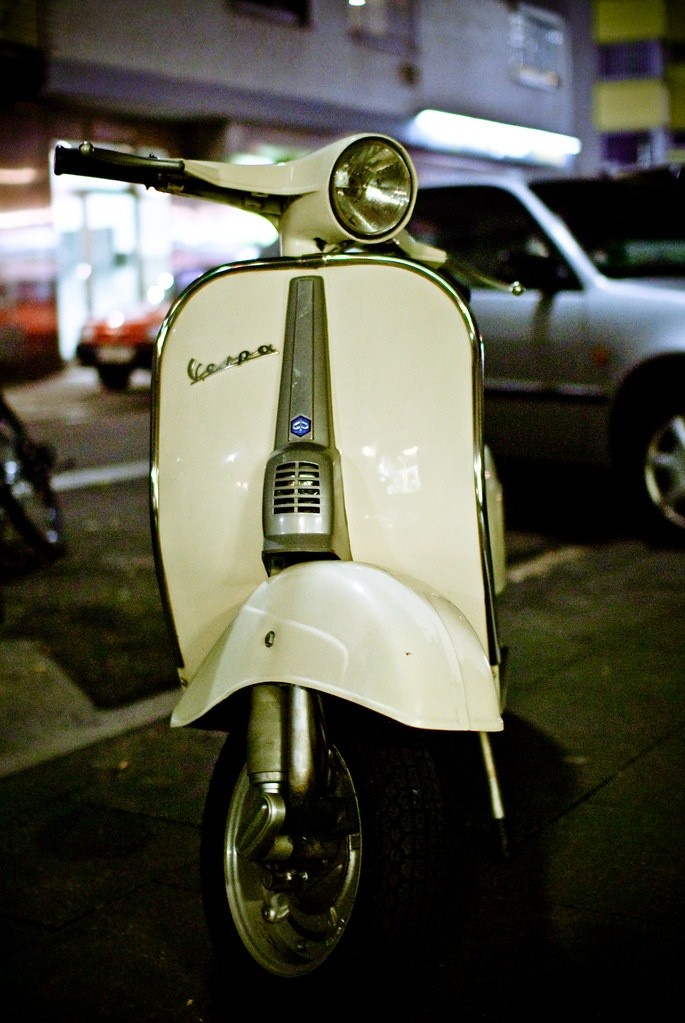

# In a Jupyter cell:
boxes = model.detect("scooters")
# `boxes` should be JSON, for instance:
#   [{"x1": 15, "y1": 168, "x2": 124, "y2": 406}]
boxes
[
  {"x1": 46, "y1": 128, "x2": 516, "y2": 1023},
  {"x1": 0, "y1": 382, "x2": 70, "y2": 569}
]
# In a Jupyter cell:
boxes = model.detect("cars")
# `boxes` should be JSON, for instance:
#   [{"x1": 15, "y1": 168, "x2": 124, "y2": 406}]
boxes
[
  {"x1": 74, "y1": 252, "x2": 247, "y2": 391},
  {"x1": 389, "y1": 161, "x2": 684, "y2": 552}
]
